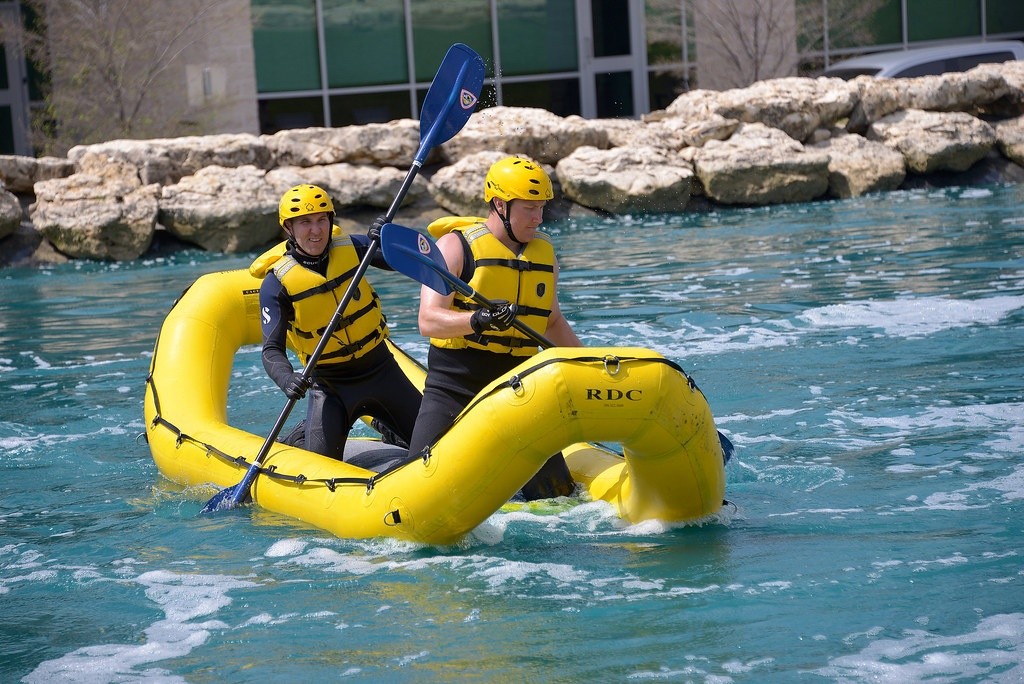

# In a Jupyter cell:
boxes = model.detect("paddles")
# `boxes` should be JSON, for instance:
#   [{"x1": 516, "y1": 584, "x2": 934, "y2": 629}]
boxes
[
  {"x1": 204, "y1": 42, "x2": 487, "y2": 510},
  {"x1": 380, "y1": 222, "x2": 736, "y2": 467}
]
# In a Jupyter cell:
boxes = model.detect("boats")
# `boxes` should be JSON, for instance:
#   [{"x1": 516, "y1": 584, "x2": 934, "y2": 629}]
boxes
[{"x1": 144, "y1": 265, "x2": 724, "y2": 554}]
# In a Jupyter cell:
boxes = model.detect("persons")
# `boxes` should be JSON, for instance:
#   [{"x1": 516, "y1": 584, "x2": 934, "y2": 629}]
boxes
[
  {"x1": 259, "y1": 184, "x2": 422, "y2": 459},
  {"x1": 406, "y1": 155, "x2": 589, "y2": 502}
]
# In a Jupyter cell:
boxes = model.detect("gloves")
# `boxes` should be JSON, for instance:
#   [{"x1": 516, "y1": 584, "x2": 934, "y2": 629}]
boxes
[
  {"x1": 281, "y1": 372, "x2": 313, "y2": 403},
  {"x1": 366, "y1": 215, "x2": 392, "y2": 242},
  {"x1": 470, "y1": 302, "x2": 517, "y2": 337}
]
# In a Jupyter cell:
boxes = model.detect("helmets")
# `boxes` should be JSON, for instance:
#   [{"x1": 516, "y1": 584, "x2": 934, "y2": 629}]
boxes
[
  {"x1": 483, "y1": 155, "x2": 554, "y2": 203},
  {"x1": 278, "y1": 184, "x2": 336, "y2": 227}
]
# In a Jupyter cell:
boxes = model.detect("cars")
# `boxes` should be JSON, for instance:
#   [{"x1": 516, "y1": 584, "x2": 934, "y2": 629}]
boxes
[{"x1": 811, "y1": 40, "x2": 1024, "y2": 78}]
